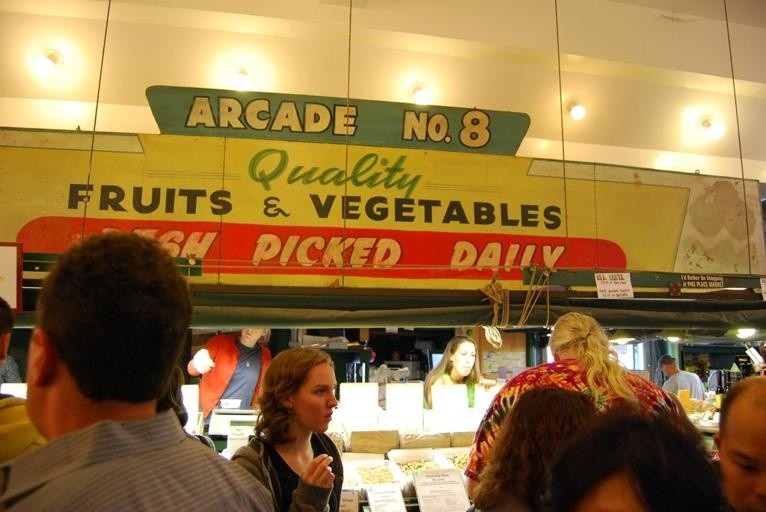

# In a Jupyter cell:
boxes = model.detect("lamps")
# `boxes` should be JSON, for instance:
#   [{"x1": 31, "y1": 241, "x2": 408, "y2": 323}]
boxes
[
  {"x1": 608, "y1": 328, "x2": 636, "y2": 345},
  {"x1": 655, "y1": 329, "x2": 690, "y2": 343},
  {"x1": 723, "y1": 321, "x2": 766, "y2": 342}
]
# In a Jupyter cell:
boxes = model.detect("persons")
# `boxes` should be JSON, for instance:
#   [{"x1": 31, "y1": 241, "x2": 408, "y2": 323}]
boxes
[
  {"x1": 156, "y1": 364, "x2": 215, "y2": 453},
  {"x1": 230, "y1": 346, "x2": 345, "y2": 512},
  {"x1": 187, "y1": 328, "x2": 272, "y2": 433},
  {"x1": 711, "y1": 375, "x2": 765, "y2": 511},
  {"x1": 0, "y1": 230, "x2": 274, "y2": 511},
  {"x1": 0, "y1": 298, "x2": 47, "y2": 468},
  {"x1": 656, "y1": 355, "x2": 705, "y2": 400},
  {"x1": 464, "y1": 313, "x2": 686, "y2": 498},
  {"x1": 548, "y1": 402, "x2": 719, "y2": 512},
  {"x1": 423, "y1": 336, "x2": 495, "y2": 409},
  {"x1": 475, "y1": 385, "x2": 596, "y2": 512}
]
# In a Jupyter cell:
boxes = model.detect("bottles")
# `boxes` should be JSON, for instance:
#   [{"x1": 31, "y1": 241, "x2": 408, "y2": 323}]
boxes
[{"x1": 715, "y1": 370, "x2": 731, "y2": 412}]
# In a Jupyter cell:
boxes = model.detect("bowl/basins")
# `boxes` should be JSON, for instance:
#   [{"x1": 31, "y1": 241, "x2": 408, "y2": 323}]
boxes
[{"x1": 219, "y1": 398, "x2": 242, "y2": 409}]
